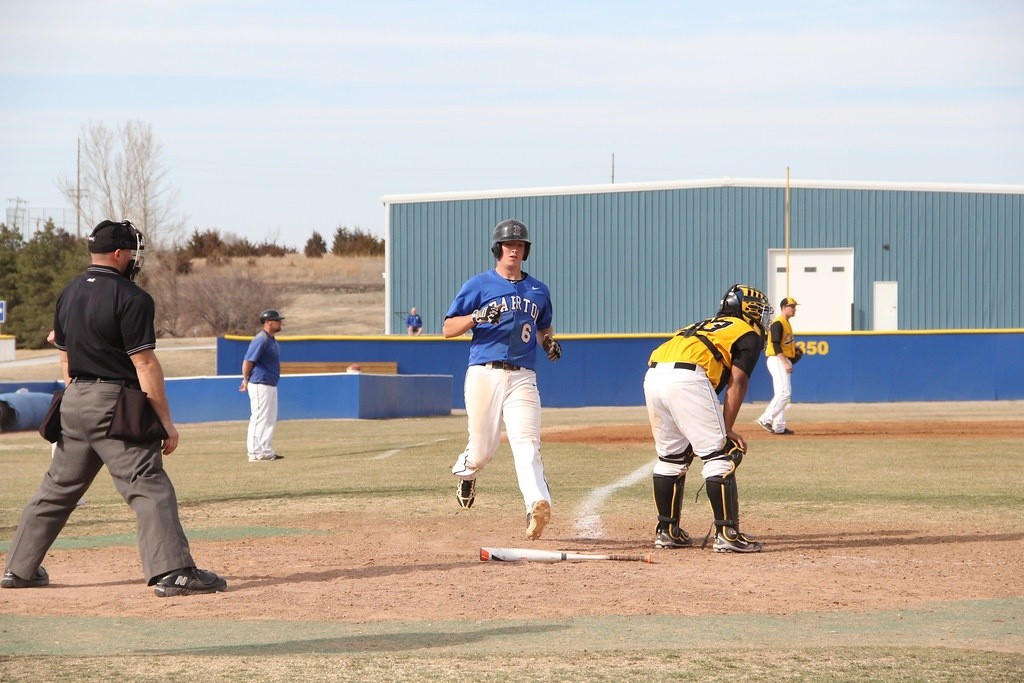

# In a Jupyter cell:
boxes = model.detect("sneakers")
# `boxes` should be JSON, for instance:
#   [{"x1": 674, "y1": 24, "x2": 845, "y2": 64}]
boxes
[
  {"x1": 757, "y1": 419, "x2": 774, "y2": 433},
  {"x1": 655, "y1": 530, "x2": 692, "y2": 548},
  {"x1": 713, "y1": 533, "x2": 762, "y2": 553},
  {"x1": 776, "y1": 428, "x2": 795, "y2": 435},
  {"x1": 154, "y1": 568, "x2": 228, "y2": 597},
  {"x1": 457, "y1": 478, "x2": 475, "y2": 510},
  {"x1": 0, "y1": 566, "x2": 49, "y2": 587},
  {"x1": 525, "y1": 500, "x2": 550, "y2": 541}
]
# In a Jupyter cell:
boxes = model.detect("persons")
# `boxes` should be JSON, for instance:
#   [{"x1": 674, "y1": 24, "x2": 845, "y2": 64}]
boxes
[
  {"x1": 406, "y1": 307, "x2": 424, "y2": 336},
  {"x1": 755, "y1": 296, "x2": 801, "y2": 437},
  {"x1": 238, "y1": 310, "x2": 285, "y2": 461},
  {"x1": 0, "y1": 219, "x2": 228, "y2": 597},
  {"x1": 442, "y1": 220, "x2": 561, "y2": 541},
  {"x1": 643, "y1": 283, "x2": 773, "y2": 554}
]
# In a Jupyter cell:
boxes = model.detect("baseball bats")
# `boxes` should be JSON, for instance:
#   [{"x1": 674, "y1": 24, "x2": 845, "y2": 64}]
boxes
[{"x1": 479, "y1": 546, "x2": 653, "y2": 564}]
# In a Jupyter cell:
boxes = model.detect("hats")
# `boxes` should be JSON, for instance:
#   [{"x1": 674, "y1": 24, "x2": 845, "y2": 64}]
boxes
[
  {"x1": 780, "y1": 297, "x2": 801, "y2": 306},
  {"x1": 89, "y1": 220, "x2": 137, "y2": 251}
]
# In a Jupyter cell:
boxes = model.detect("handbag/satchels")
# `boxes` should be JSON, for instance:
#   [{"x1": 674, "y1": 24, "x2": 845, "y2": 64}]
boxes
[
  {"x1": 39, "y1": 390, "x2": 62, "y2": 443},
  {"x1": 106, "y1": 384, "x2": 169, "y2": 441}
]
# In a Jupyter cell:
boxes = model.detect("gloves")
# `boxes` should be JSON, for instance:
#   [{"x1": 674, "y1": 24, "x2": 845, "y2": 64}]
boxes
[
  {"x1": 542, "y1": 334, "x2": 562, "y2": 361},
  {"x1": 472, "y1": 304, "x2": 502, "y2": 325}
]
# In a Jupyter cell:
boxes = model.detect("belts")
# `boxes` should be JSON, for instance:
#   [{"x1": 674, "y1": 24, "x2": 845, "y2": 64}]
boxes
[
  {"x1": 649, "y1": 362, "x2": 696, "y2": 371},
  {"x1": 77, "y1": 376, "x2": 122, "y2": 384},
  {"x1": 478, "y1": 362, "x2": 520, "y2": 370}
]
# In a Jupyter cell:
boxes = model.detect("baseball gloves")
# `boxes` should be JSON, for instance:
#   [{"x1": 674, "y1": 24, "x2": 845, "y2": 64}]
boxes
[{"x1": 787, "y1": 345, "x2": 804, "y2": 364}]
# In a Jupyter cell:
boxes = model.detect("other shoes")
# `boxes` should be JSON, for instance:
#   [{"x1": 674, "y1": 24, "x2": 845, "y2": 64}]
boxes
[{"x1": 274, "y1": 455, "x2": 285, "y2": 460}]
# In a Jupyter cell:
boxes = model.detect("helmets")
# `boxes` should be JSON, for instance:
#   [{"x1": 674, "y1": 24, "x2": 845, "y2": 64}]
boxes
[
  {"x1": 260, "y1": 309, "x2": 285, "y2": 320},
  {"x1": 491, "y1": 219, "x2": 532, "y2": 261},
  {"x1": 722, "y1": 285, "x2": 774, "y2": 345}
]
